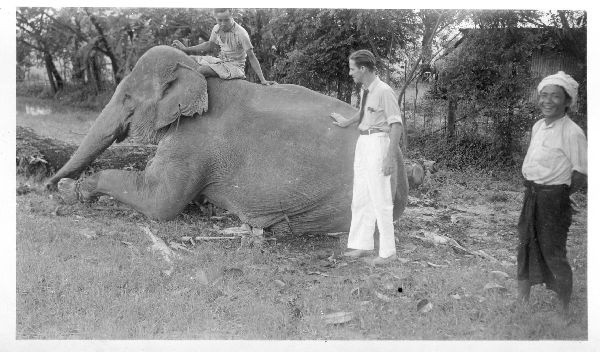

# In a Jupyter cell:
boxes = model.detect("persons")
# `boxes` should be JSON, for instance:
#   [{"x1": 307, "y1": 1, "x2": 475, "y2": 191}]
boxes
[
  {"x1": 510, "y1": 70, "x2": 587, "y2": 318},
  {"x1": 328, "y1": 50, "x2": 403, "y2": 265},
  {"x1": 170, "y1": 9, "x2": 279, "y2": 86}
]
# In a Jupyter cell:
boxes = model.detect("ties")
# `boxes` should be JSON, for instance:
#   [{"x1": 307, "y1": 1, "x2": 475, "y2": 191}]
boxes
[{"x1": 359, "y1": 89, "x2": 369, "y2": 125}]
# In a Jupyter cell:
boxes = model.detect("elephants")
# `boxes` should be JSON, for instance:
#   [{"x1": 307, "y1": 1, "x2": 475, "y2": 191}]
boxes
[{"x1": 47, "y1": 44, "x2": 409, "y2": 234}]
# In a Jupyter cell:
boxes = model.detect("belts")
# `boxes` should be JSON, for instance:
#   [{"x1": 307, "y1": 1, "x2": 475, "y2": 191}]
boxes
[{"x1": 359, "y1": 129, "x2": 381, "y2": 135}]
